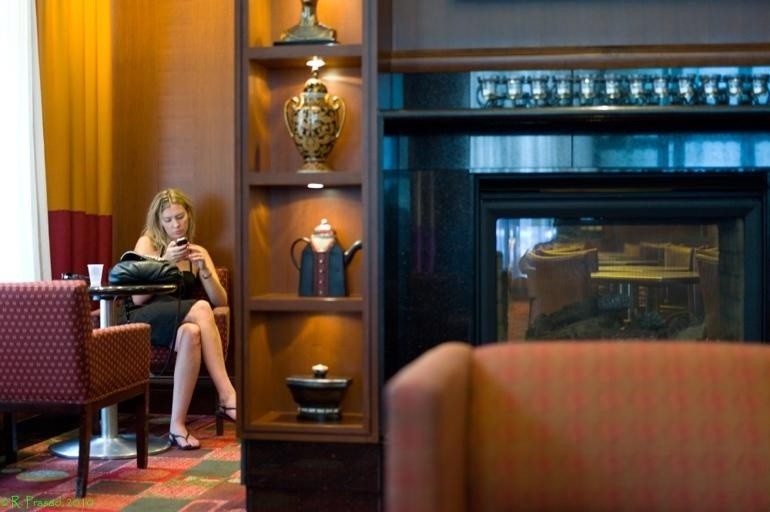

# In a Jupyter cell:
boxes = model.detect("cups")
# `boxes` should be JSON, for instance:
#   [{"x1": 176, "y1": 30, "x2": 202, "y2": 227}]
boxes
[
  {"x1": 477, "y1": 73, "x2": 770, "y2": 109},
  {"x1": 87, "y1": 264, "x2": 104, "y2": 286}
]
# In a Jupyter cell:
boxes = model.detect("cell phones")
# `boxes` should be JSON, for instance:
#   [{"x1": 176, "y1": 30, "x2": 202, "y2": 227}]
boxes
[{"x1": 176, "y1": 237, "x2": 188, "y2": 246}]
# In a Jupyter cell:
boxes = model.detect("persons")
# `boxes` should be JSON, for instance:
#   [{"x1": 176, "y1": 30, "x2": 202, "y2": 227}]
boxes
[{"x1": 117, "y1": 188, "x2": 237, "y2": 450}]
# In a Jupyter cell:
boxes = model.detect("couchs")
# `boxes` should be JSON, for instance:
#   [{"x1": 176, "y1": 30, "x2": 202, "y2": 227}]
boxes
[{"x1": 381, "y1": 339, "x2": 770, "y2": 512}]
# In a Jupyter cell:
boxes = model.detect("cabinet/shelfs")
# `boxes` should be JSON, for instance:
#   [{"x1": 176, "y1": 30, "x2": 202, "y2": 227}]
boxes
[{"x1": 235, "y1": 0, "x2": 376, "y2": 510}]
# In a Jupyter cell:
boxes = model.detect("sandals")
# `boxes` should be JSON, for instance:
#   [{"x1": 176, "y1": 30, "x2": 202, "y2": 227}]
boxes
[
  {"x1": 169, "y1": 431, "x2": 200, "y2": 450},
  {"x1": 214, "y1": 405, "x2": 237, "y2": 422}
]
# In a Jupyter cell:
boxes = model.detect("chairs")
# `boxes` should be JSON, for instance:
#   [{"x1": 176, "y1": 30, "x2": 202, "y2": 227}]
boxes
[
  {"x1": 91, "y1": 267, "x2": 230, "y2": 437},
  {"x1": 519, "y1": 239, "x2": 719, "y2": 339},
  {"x1": 0, "y1": 278, "x2": 150, "y2": 498}
]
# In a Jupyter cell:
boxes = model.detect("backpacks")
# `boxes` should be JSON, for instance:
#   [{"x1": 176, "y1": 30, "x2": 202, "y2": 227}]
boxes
[{"x1": 107, "y1": 251, "x2": 183, "y2": 285}]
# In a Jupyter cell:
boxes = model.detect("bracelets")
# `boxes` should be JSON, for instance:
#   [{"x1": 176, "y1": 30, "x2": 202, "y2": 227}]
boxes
[{"x1": 203, "y1": 271, "x2": 212, "y2": 280}]
